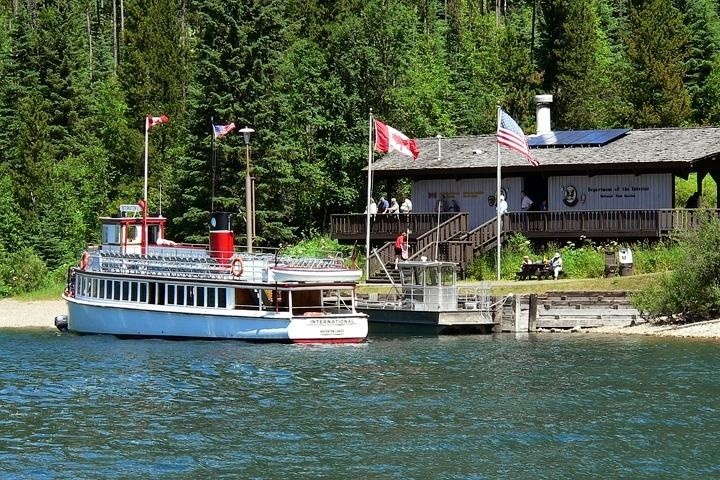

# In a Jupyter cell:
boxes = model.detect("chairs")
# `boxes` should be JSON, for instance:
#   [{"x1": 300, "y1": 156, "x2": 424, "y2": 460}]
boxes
[{"x1": 93, "y1": 249, "x2": 335, "y2": 286}]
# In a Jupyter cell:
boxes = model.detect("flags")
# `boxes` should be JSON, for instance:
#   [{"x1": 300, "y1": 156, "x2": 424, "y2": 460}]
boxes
[
  {"x1": 146, "y1": 114, "x2": 169, "y2": 131},
  {"x1": 212, "y1": 122, "x2": 236, "y2": 140},
  {"x1": 496, "y1": 109, "x2": 540, "y2": 167},
  {"x1": 373, "y1": 118, "x2": 420, "y2": 160}
]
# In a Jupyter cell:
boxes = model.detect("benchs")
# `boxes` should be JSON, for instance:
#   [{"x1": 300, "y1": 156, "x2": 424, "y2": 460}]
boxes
[{"x1": 516, "y1": 270, "x2": 566, "y2": 281}]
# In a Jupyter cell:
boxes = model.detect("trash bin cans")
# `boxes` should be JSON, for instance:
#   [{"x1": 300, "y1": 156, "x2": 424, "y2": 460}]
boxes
[
  {"x1": 604, "y1": 252, "x2": 617, "y2": 278},
  {"x1": 618, "y1": 247, "x2": 633, "y2": 276}
]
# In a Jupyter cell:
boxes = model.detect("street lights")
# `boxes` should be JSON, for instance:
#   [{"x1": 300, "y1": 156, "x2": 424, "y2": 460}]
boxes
[{"x1": 238, "y1": 126, "x2": 255, "y2": 256}]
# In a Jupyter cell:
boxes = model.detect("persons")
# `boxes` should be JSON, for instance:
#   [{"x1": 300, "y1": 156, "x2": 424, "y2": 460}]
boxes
[
  {"x1": 364, "y1": 196, "x2": 412, "y2": 214},
  {"x1": 540, "y1": 199, "x2": 547, "y2": 211},
  {"x1": 521, "y1": 190, "x2": 533, "y2": 211},
  {"x1": 549, "y1": 253, "x2": 562, "y2": 280},
  {"x1": 394, "y1": 232, "x2": 407, "y2": 270},
  {"x1": 520, "y1": 255, "x2": 533, "y2": 273},
  {"x1": 447, "y1": 200, "x2": 460, "y2": 212},
  {"x1": 80, "y1": 251, "x2": 89, "y2": 271},
  {"x1": 496, "y1": 194, "x2": 508, "y2": 216},
  {"x1": 435, "y1": 194, "x2": 447, "y2": 212}
]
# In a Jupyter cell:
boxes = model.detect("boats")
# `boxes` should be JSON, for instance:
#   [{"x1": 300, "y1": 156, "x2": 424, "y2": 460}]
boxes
[
  {"x1": 277, "y1": 200, "x2": 500, "y2": 336},
  {"x1": 62, "y1": 114, "x2": 369, "y2": 344},
  {"x1": 268, "y1": 252, "x2": 365, "y2": 283}
]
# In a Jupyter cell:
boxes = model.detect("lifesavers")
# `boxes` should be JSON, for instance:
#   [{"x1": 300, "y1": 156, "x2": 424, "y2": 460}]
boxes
[
  {"x1": 80, "y1": 253, "x2": 87, "y2": 270},
  {"x1": 232, "y1": 258, "x2": 242, "y2": 277}
]
